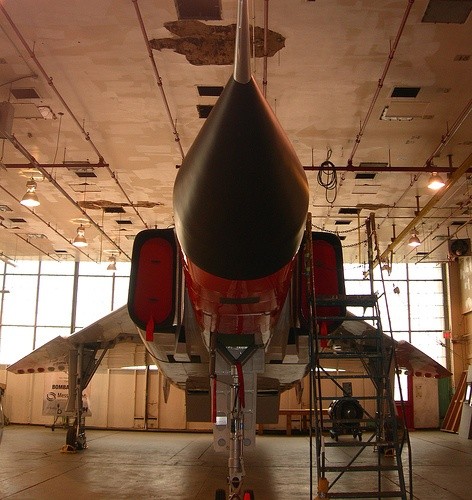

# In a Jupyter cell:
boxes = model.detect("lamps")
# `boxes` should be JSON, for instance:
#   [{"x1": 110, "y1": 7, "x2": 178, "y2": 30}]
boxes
[
  {"x1": 72, "y1": 224, "x2": 88, "y2": 247},
  {"x1": 408, "y1": 227, "x2": 421, "y2": 246},
  {"x1": 19, "y1": 173, "x2": 40, "y2": 207},
  {"x1": 106, "y1": 255, "x2": 117, "y2": 271},
  {"x1": 427, "y1": 172, "x2": 445, "y2": 190}
]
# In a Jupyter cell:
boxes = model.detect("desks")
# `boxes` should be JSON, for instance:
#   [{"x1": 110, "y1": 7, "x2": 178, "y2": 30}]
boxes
[{"x1": 258, "y1": 409, "x2": 330, "y2": 437}]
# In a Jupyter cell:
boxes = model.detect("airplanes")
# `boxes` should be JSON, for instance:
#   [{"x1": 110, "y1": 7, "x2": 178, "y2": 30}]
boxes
[{"x1": 3, "y1": 0, "x2": 455, "y2": 499}]
